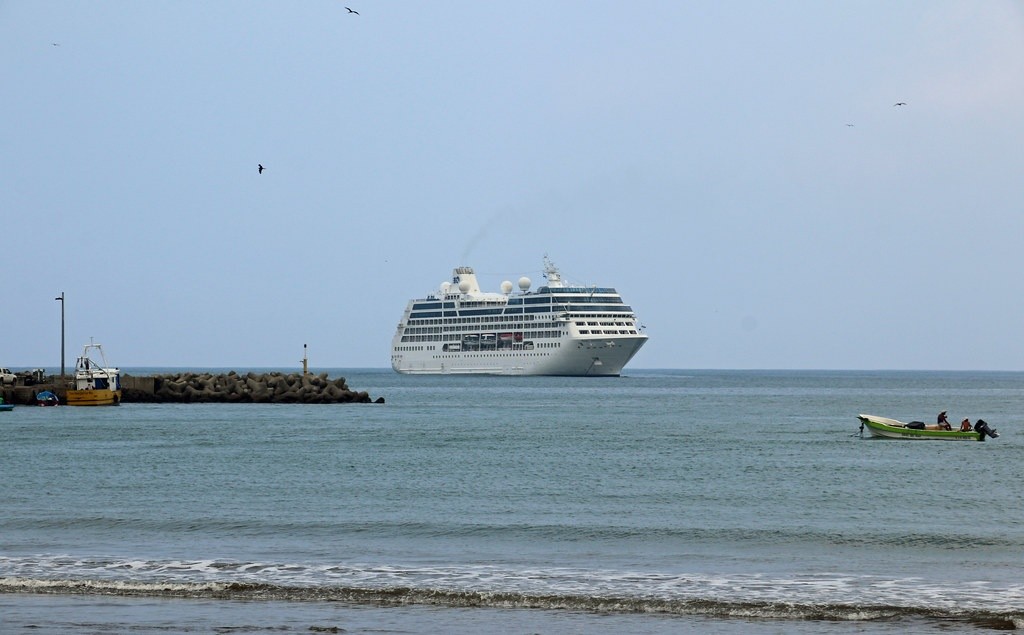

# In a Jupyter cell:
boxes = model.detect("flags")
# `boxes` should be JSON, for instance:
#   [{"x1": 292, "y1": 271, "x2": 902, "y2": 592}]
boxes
[{"x1": 543, "y1": 273, "x2": 546, "y2": 278}]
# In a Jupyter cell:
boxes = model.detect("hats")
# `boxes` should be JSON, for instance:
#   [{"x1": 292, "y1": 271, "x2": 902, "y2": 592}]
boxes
[{"x1": 964, "y1": 417, "x2": 968, "y2": 420}]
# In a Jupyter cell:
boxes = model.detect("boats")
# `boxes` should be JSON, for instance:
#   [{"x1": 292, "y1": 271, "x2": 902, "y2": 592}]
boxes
[
  {"x1": 499, "y1": 334, "x2": 512, "y2": 340},
  {"x1": 66, "y1": 335, "x2": 122, "y2": 406},
  {"x1": 856, "y1": 414, "x2": 1000, "y2": 441},
  {"x1": 0, "y1": 398, "x2": 14, "y2": 411},
  {"x1": 36, "y1": 391, "x2": 59, "y2": 407}
]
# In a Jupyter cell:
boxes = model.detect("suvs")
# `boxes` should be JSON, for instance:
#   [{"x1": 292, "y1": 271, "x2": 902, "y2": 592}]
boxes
[{"x1": 0, "y1": 368, "x2": 18, "y2": 387}]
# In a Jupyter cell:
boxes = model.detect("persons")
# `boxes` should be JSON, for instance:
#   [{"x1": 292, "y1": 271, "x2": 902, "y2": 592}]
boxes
[
  {"x1": 960, "y1": 418, "x2": 972, "y2": 432},
  {"x1": 937, "y1": 411, "x2": 951, "y2": 431}
]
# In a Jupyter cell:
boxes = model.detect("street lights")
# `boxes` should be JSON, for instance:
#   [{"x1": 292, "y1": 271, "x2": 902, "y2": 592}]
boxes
[{"x1": 54, "y1": 297, "x2": 64, "y2": 375}]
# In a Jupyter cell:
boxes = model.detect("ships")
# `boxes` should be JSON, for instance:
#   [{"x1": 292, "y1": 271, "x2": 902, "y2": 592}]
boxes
[{"x1": 390, "y1": 254, "x2": 649, "y2": 377}]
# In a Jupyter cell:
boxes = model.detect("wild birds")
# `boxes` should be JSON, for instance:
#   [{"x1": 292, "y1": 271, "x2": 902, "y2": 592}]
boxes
[
  {"x1": 894, "y1": 103, "x2": 906, "y2": 106},
  {"x1": 258, "y1": 164, "x2": 265, "y2": 174},
  {"x1": 847, "y1": 124, "x2": 853, "y2": 126},
  {"x1": 345, "y1": 7, "x2": 359, "y2": 15}
]
